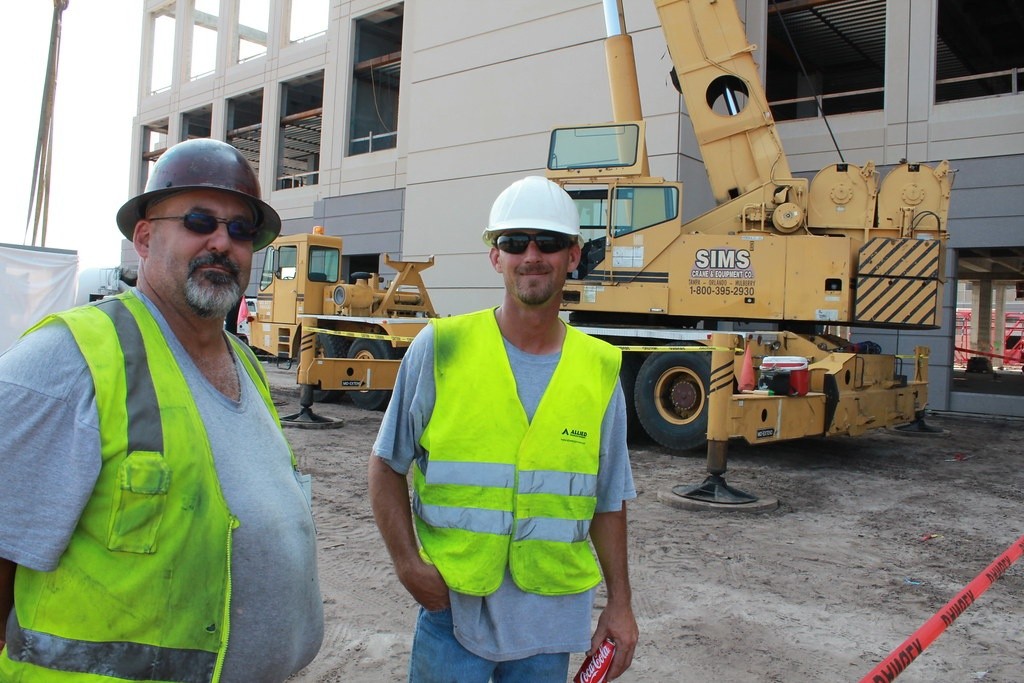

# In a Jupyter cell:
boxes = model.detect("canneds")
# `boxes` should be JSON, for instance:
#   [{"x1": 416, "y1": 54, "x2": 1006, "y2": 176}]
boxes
[{"x1": 573, "y1": 637, "x2": 617, "y2": 683}]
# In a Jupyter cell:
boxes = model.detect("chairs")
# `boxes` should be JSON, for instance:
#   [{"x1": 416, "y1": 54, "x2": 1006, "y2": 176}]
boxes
[{"x1": 309, "y1": 272, "x2": 326, "y2": 282}]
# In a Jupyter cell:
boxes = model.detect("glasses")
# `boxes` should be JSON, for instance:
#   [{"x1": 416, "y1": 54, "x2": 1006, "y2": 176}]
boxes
[
  {"x1": 146, "y1": 211, "x2": 260, "y2": 242},
  {"x1": 490, "y1": 232, "x2": 572, "y2": 254}
]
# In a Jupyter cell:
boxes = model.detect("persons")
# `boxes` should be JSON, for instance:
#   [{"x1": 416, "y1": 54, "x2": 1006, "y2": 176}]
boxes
[
  {"x1": 367, "y1": 176, "x2": 640, "y2": 683},
  {"x1": 0, "y1": 137, "x2": 325, "y2": 683}
]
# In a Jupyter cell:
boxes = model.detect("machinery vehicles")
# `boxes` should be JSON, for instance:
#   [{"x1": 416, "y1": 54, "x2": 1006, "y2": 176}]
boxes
[{"x1": 224, "y1": 1, "x2": 961, "y2": 515}]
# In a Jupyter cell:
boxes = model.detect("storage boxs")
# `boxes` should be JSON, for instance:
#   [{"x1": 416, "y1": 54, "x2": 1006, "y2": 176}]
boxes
[{"x1": 759, "y1": 355, "x2": 808, "y2": 396}]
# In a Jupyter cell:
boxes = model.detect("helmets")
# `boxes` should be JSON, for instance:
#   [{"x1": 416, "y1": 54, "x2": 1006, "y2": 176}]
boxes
[
  {"x1": 482, "y1": 175, "x2": 585, "y2": 249},
  {"x1": 115, "y1": 138, "x2": 282, "y2": 253}
]
